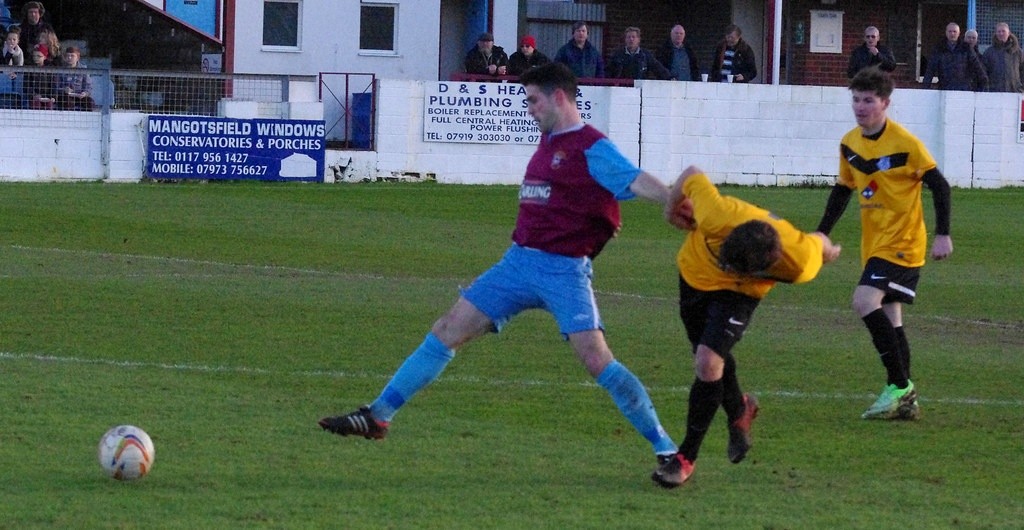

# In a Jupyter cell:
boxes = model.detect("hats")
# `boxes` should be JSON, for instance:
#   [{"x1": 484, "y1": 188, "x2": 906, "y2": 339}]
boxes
[
  {"x1": 478, "y1": 32, "x2": 494, "y2": 42},
  {"x1": 32, "y1": 43, "x2": 50, "y2": 57},
  {"x1": 519, "y1": 35, "x2": 535, "y2": 49}
]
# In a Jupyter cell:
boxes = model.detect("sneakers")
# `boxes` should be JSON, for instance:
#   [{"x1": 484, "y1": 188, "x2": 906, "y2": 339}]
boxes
[
  {"x1": 728, "y1": 391, "x2": 760, "y2": 465},
  {"x1": 650, "y1": 451, "x2": 677, "y2": 481},
  {"x1": 318, "y1": 405, "x2": 387, "y2": 440},
  {"x1": 861, "y1": 376, "x2": 919, "y2": 419},
  {"x1": 652, "y1": 450, "x2": 694, "y2": 487}
]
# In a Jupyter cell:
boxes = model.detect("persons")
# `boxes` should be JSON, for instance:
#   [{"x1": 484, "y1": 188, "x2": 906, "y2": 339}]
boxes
[
  {"x1": 922, "y1": 23, "x2": 1024, "y2": 93},
  {"x1": 318, "y1": 60, "x2": 681, "y2": 479},
  {"x1": 0, "y1": 0, "x2": 96, "y2": 110},
  {"x1": 816, "y1": 67, "x2": 952, "y2": 419},
  {"x1": 846, "y1": 25, "x2": 896, "y2": 83},
  {"x1": 465, "y1": 23, "x2": 758, "y2": 82},
  {"x1": 651, "y1": 165, "x2": 841, "y2": 488}
]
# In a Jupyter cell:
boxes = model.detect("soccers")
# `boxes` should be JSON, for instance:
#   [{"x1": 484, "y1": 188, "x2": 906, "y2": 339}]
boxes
[{"x1": 97, "y1": 424, "x2": 155, "y2": 481}]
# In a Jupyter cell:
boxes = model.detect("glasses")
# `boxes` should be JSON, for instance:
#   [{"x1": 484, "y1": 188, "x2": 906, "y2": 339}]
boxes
[
  {"x1": 866, "y1": 35, "x2": 876, "y2": 39},
  {"x1": 521, "y1": 44, "x2": 531, "y2": 47}
]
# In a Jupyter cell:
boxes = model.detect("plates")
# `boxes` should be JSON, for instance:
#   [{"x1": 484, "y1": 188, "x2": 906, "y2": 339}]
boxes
[
  {"x1": 39, "y1": 98, "x2": 51, "y2": 102},
  {"x1": 69, "y1": 93, "x2": 81, "y2": 97}
]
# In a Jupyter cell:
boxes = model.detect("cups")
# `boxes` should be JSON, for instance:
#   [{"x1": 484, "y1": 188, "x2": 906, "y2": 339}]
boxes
[
  {"x1": 727, "y1": 75, "x2": 734, "y2": 83},
  {"x1": 701, "y1": 74, "x2": 708, "y2": 81}
]
